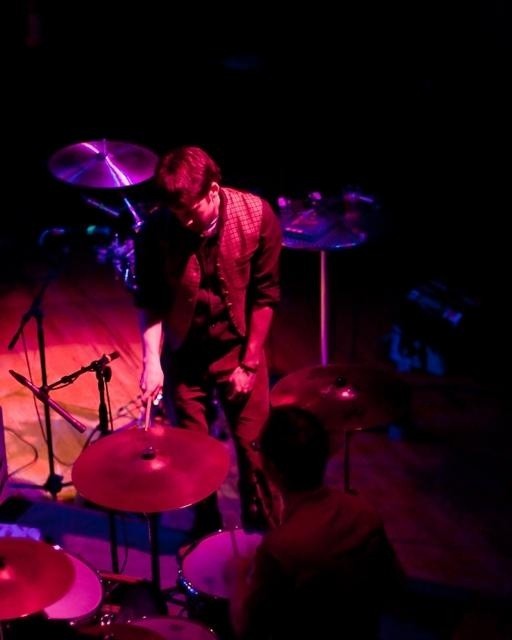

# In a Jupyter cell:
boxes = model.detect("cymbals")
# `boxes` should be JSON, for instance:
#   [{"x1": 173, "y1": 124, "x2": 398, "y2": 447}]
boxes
[
  {"x1": 269, "y1": 362, "x2": 414, "y2": 431},
  {"x1": 49, "y1": 139, "x2": 157, "y2": 190},
  {"x1": 69, "y1": 428, "x2": 232, "y2": 514},
  {"x1": 268, "y1": 190, "x2": 373, "y2": 249},
  {"x1": 0, "y1": 535, "x2": 75, "y2": 619}
]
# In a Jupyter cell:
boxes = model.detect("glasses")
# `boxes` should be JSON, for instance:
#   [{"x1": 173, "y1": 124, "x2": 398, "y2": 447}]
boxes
[{"x1": 249, "y1": 438, "x2": 262, "y2": 451}]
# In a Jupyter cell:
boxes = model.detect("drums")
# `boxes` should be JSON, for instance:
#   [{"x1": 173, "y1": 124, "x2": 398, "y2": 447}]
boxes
[
  {"x1": 15, "y1": 542, "x2": 104, "y2": 640},
  {"x1": 99, "y1": 614, "x2": 218, "y2": 636},
  {"x1": 180, "y1": 527, "x2": 267, "y2": 640}
]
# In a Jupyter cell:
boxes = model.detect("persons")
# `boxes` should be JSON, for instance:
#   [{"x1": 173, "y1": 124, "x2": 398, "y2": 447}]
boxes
[
  {"x1": 230, "y1": 402, "x2": 406, "y2": 639},
  {"x1": 134, "y1": 145, "x2": 283, "y2": 548}
]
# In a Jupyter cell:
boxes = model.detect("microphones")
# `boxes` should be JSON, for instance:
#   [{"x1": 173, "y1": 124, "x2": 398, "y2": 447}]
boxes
[
  {"x1": 308, "y1": 192, "x2": 322, "y2": 200},
  {"x1": 8, "y1": 274, "x2": 52, "y2": 350},
  {"x1": 277, "y1": 196, "x2": 304, "y2": 208},
  {"x1": 8, "y1": 370, "x2": 86, "y2": 434}
]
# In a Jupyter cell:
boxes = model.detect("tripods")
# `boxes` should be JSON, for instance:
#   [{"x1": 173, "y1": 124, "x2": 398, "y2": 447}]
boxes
[{"x1": 4, "y1": 310, "x2": 74, "y2": 502}]
